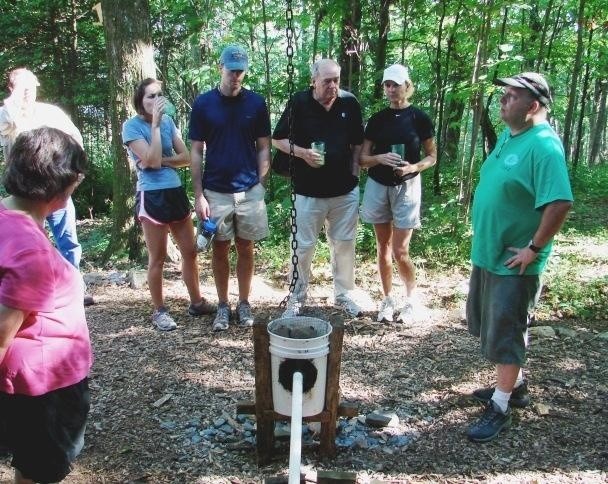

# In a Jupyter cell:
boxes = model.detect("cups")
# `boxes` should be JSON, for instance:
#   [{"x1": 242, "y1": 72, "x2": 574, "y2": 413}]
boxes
[
  {"x1": 390, "y1": 144, "x2": 404, "y2": 172},
  {"x1": 311, "y1": 142, "x2": 324, "y2": 165},
  {"x1": 154, "y1": 97, "x2": 176, "y2": 116}
]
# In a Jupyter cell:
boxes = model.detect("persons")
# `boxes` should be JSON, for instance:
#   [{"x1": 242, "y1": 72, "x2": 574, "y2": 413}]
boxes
[
  {"x1": 0, "y1": 66, "x2": 94, "y2": 307},
  {"x1": 463, "y1": 71, "x2": 573, "y2": 444},
  {"x1": 365, "y1": 64, "x2": 439, "y2": 324},
  {"x1": 121, "y1": 77, "x2": 219, "y2": 332},
  {"x1": 187, "y1": 44, "x2": 272, "y2": 331},
  {"x1": 270, "y1": 57, "x2": 365, "y2": 319},
  {"x1": 0, "y1": 126, "x2": 94, "y2": 483}
]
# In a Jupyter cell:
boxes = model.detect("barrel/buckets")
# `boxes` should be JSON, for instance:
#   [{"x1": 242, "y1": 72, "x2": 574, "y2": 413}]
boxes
[{"x1": 267, "y1": 315, "x2": 333, "y2": 417}]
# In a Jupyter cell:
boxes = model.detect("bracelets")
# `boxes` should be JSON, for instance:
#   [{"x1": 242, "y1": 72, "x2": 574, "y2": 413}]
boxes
[{"x1": 528, "y1": 239, "x2": 541, "y2": 253}]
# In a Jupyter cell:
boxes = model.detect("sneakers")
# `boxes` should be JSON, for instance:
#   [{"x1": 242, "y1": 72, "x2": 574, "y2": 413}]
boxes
[
  {"x1": 377, "y1": 299, "x2": 398, "y2": 322},
  {"x1": 472, "y1": 381, "x2": 531, "y2": 406},
  {"x1": 189, "y1": 298, "x2": 217, "y2": 317},
  {"x1": 335, "y1": 294, "x2": 364, "y2": 317},
  {"x1": 236, "y1": 300, "x2": 255, "y2": 325},
  {"x1": 282, "y1": 302, "x2": 305, "y2": 318},
  {"x1": 152, "y1": 307, "x2": 177, "y2": 331},
  {"x1": 397, "y1": 304, "x2": 416, "y2": 326},
  {"x1": 465, "y1": 400, "x2": 514, "y2": 443},
  {"x1": 211, "y1": 303, "x2": 231, "y2": 329}
]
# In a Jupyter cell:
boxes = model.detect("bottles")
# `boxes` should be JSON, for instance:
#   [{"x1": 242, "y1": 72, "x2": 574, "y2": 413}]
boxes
[{"x1": 193, "y1": 217, "x2": 216, "y2": 254}]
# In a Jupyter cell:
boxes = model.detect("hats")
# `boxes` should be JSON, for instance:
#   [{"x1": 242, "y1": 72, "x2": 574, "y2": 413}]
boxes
[
  {"x1": 381, "y1": 64, "x2": 409, "y2": 86},
  {"x1": 220, "y1": 47, "x2": 249, "y2": 73},
  {"x1": 492, "y1": 72, "x2": 551, "y2": 105}
]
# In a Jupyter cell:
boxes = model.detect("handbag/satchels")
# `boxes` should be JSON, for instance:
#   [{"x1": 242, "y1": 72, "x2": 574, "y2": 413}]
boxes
[{"x1": 272, "y1": 150, "x2": 291, "y2": 178}]
